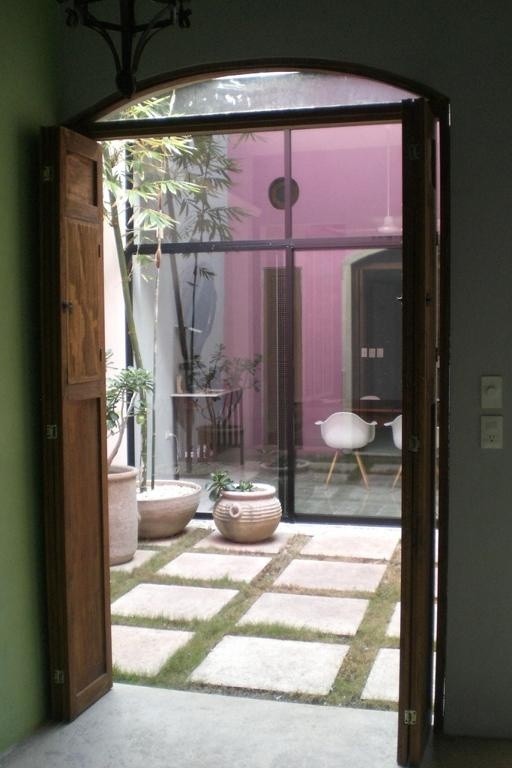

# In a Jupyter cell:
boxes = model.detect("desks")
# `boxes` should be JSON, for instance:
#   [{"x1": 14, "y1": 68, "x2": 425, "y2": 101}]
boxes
[{"x1": 170, "y1": 387, "x2": 243, "y2": 481}]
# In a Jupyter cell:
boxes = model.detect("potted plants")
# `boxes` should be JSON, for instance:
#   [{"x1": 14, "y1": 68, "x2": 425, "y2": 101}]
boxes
[
  {"x1": 205, "y1": 470, "x2": 283, "y2": 543},
  {"x1": 105, "y1": 349, "x2": 156, "y2": 567},
  {"x1": 101, "y1": 96, "x2": 206, "y2": 540}
]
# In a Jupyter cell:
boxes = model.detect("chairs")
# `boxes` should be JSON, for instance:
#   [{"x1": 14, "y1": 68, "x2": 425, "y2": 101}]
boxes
[
  {"x1": 315, "y1": 412, "x2": 378, "y2": 488},
  {"x1": 390, "y1": 415, "x2": 439, "y2": 490}
]
